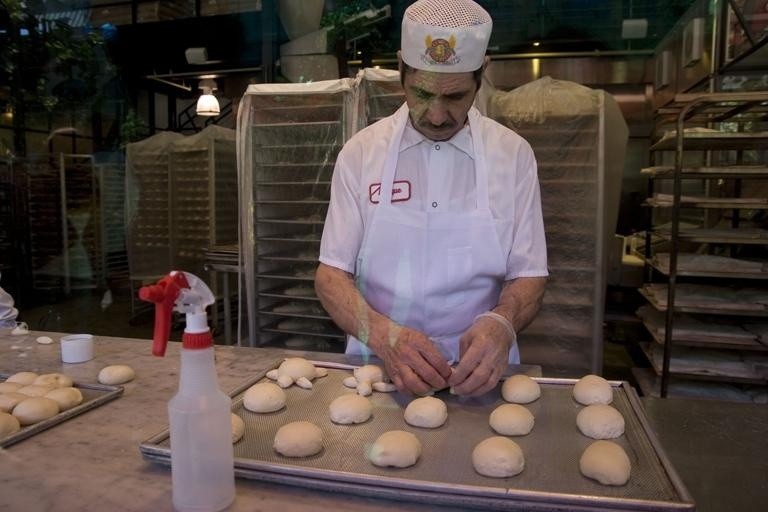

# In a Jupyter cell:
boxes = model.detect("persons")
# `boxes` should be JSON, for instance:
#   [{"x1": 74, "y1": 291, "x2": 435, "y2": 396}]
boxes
[{"x1": 313, "y1": 0, "x2": 549, "y2": 398}]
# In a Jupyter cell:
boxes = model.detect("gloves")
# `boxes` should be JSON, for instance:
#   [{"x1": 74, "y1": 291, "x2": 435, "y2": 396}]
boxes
[{"x1": 448, "y1": 309, "x2": 520, "y2": 400}]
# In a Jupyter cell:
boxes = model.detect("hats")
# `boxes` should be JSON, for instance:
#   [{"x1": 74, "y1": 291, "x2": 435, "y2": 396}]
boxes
[{"x1": 400, "y1": 0, "x2": 493, "y2": 73}]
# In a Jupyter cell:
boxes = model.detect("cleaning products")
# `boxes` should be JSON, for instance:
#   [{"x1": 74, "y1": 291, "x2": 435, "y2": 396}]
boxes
[{"x1": 139, "y1": 270, "x2": 236, "y2": 512}]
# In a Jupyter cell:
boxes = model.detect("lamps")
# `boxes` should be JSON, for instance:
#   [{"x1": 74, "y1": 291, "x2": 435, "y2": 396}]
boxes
[{"x1": 194, "y1": 78, "x2": 222, "y2": 118}]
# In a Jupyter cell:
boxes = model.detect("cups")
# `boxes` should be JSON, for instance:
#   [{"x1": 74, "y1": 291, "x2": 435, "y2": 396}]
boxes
[{"x1": 60, "y1": 333, "x2": 96, "y2": 363}]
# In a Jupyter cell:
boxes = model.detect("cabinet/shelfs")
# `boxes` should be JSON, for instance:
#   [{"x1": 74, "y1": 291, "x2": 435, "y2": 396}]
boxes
[
  {"x1": 356, "y1": 70, "x2": 408, "y2": 132},
  {"x1": 486, "y1": 85, "x2": 604, "y2": 377},
  {"x1": 237, "y1": 80, "x2": 348, "y2": 351},
  {"x1": 633, "y1": 92, "x2": 768, "y2": 398}
]
